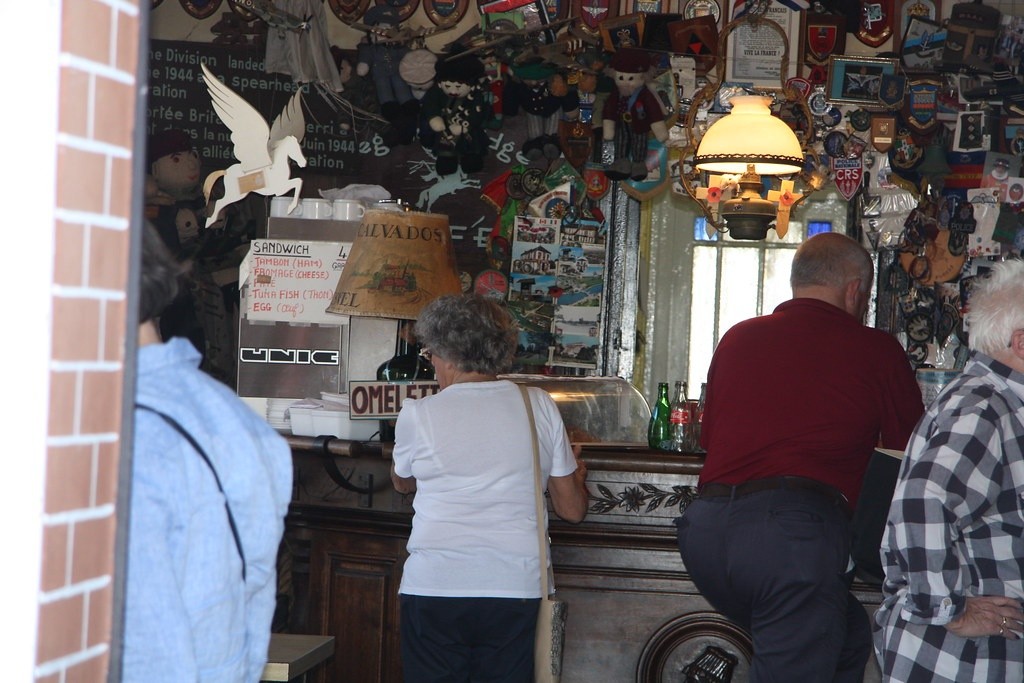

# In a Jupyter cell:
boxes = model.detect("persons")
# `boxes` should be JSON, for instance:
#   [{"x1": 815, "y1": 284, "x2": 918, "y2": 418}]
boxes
[
  {"x1": 980, "y1": 158, "x2": 1009, "y2": 202},
  {"x1": 871, "y1": 258, "x2": 1024, "y2": 683},
  {"x1": 355, "y1": 5, "x2": 670, "y2": 180},
  {"x1": 146, "y1": 129, "x2": 230, "y2": 256},
  {"x1": 676, "y1": 233, "x2": 925, "y2": 683},
  {"x1": 392, "y1": 294, "x2": 588, "y2": 683},
  {"x1": 119, "y1": 255, "x2": 293, "y2": 683}
]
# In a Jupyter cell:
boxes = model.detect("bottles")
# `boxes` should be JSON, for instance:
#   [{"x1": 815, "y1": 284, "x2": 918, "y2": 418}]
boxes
[
  {"x1": 672, "y1": 382, "x2": 692, "y2": 451},
  {"x1": 693, "y1": 383, "x2": 707, "y2": 453},
  {"x1": 671, "y1": 382, "x2": 681, "y2": 407},
  {"x1": 647, "y1": 382, "x2": 671, "y2": 450}
]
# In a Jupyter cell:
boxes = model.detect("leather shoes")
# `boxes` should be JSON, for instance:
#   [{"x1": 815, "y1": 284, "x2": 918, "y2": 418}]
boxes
[{"x1": 698, "y1": 474, "x2": 841, "y2": 498}]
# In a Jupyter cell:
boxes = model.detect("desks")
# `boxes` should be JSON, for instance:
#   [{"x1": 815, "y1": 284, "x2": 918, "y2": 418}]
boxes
[{"x1": 259, "y1": 633, "x2": 336, "y2": 683}]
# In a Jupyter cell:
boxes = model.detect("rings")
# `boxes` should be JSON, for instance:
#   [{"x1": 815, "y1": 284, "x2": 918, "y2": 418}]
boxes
[
  {"x1": 1001, "y1": 616, "x2": 1006, "y2": 627},
  {"x1": 999, "y1": 627, "x2": 1004, "y2": 637}
]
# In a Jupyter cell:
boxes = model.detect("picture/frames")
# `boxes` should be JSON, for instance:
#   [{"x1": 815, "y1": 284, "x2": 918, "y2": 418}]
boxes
[{"x1": 824, "y1": 54, "x2": 901, "y2": 109}]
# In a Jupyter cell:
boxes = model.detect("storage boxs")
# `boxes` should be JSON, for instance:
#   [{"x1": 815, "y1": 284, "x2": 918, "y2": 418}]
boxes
[{"x1": 290, "y1": 407, "x2": 380, "y2": 442}]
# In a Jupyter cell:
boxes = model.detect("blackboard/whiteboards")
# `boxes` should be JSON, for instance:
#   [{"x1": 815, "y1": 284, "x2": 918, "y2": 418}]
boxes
[{"x1": 147, "y1": 38, "x2": 365, "y2": 179}]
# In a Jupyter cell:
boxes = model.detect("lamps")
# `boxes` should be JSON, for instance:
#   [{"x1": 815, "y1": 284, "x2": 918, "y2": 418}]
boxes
[
  {"x1": 324, "y1": 209, "x2": 464, "y2": 443},
  {"x1": 692, "y1": 96, "x2": 804, "y2": 241}
]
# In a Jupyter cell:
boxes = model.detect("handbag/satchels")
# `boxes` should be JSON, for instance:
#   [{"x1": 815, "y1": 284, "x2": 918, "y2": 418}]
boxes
[{"x1": 533, "y1": 598, "x2": 569, "y2": 683}]
